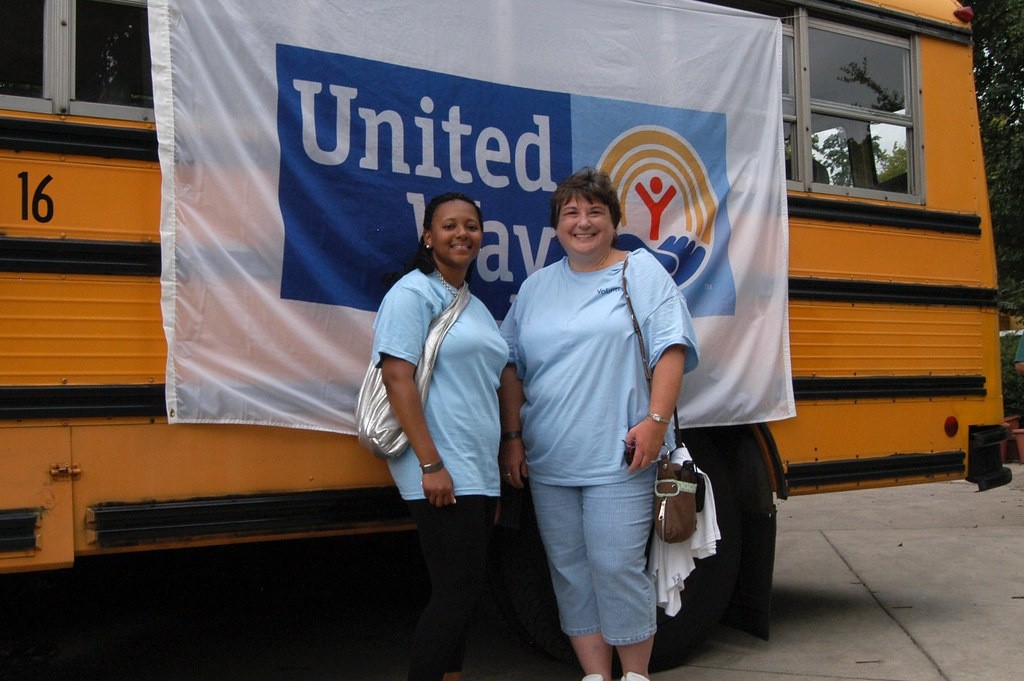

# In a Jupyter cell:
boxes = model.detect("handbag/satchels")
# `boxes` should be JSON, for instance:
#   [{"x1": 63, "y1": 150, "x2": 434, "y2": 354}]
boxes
[
  {"x1": 355, "y1": 281, "x2": 471, "y2": 461},
  {"x1": 654, "y1": 442, "x2": 698, "y2": 544}
]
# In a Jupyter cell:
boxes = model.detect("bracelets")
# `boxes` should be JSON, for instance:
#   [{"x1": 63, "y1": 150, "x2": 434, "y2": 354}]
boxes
[
  {"x1": 504, "y1": 431, "x2": 522, "y2": 438},
  {"x1": 422, "y1": 459, "x2": 444, "y2": 473}
]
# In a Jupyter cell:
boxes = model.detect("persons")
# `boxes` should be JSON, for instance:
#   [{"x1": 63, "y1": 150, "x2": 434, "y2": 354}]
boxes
[
  {"x1": 1014, "y1": 332, "x2": 1024, "y2": 373},
  {"x1": 499, "y1": 168, "x2": 700, "y2": 681},
  {"x1": 372, "y1": 194, "x2": 510, "y2": 681}
]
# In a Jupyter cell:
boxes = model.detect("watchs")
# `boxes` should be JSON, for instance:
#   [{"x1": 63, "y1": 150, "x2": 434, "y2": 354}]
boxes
[{"x1": 647, "y1": 412, "x2": 670, "y2": 423}]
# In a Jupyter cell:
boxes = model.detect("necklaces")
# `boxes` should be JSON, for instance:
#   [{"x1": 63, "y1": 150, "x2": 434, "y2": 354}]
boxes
[{"x1": 436, "y1": 271, "x2": 457, "y2": 297}]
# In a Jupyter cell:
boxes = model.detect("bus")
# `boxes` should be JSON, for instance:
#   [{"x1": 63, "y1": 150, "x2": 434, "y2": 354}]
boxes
[{"x1": 0, "y1": 0, "x2": 1012, "y2": 674}]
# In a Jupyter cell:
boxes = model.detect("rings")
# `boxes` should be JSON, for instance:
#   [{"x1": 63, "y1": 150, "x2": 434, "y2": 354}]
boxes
[
  {"x1": 503, "y1": 474, "x2": 512, "y2": 477},
  {"x1": 649, "y1": 460, "x2": 654, "y2": 465}
]
